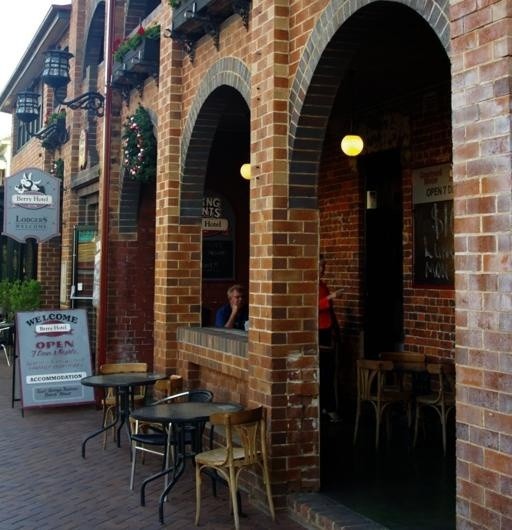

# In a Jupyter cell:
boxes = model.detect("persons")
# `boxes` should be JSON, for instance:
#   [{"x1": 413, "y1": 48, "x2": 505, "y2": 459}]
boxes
[
  {"x1": 214, "y1": 284, "x2": 249, "y2": 330},
  {"x1": 317, "y1": 253, "x2": 348, "y2": 427}
]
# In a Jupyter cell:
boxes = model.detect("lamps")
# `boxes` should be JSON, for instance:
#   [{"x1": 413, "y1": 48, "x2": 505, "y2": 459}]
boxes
[
  {"x1": 340, "y1": 117, "x2": 364, "y2": 157},
  {"x1": 42, "y1": 43, "x2": 104, "y2": 117},
  {"x1": 16, "y1": 87, "x2": 70, "y2": 154},
  {"x1": 240, "y1": 163, "x2": 253, "y2": 180}
]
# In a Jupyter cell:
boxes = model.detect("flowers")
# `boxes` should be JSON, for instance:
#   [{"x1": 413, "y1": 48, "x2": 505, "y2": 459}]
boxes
[
  {"x1": 120, "y1": 105, "x2": 158, "y2": 180},
  {"x1": 109, "y1": 23, "x2": 159, "y2": 62}
]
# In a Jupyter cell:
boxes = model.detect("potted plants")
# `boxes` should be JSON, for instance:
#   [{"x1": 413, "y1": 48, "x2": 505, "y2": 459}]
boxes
[{"x1": 168, "y1": 0, "x2": 251, "y2": 63}]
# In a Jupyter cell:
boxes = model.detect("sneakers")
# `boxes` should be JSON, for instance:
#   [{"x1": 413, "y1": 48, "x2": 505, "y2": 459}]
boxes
[{"x1": 323, "y1": 410, "x2": 339, "y2": 422}]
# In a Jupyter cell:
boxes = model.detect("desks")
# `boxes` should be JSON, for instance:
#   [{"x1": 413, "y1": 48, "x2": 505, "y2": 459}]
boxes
[{"x1": 81, "y1": 373, "x2": 162, "y2": 458}]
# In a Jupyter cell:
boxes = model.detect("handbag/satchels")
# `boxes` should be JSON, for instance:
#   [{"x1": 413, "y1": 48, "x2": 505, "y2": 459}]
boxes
[{"x1": 319, "y1": 316, "x2": 340, "y2": 347}]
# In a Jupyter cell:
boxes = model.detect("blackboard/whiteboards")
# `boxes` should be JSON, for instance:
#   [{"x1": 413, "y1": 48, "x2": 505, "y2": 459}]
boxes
[
  {"x1": 412, "y1": 200, "x2": 453, "y2": 291},
  {"x1": 201, "y1": 190, "x2": 236, "y2": 281}
]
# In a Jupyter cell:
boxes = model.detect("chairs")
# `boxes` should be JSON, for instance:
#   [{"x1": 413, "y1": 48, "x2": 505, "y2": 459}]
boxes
[
  {"x1": 100, "y1": 363, "x2": 275, "y2": 530},
  {"x1": 353, "y1": 352, "x2": 455, "y2": 456}
]
